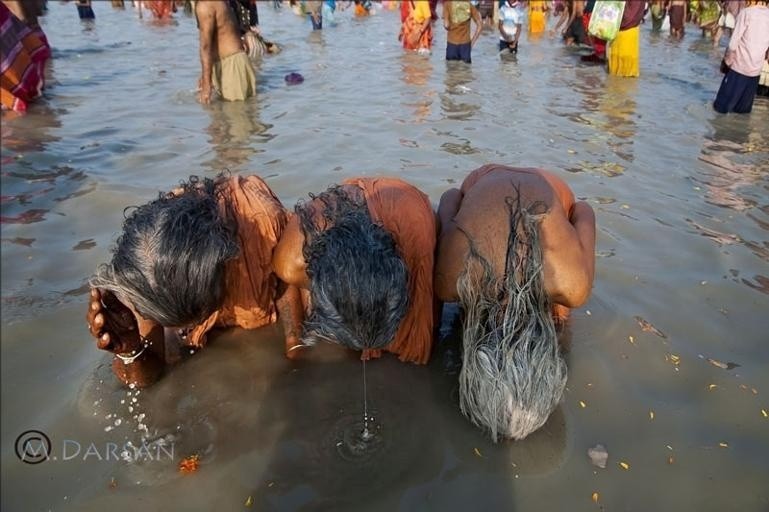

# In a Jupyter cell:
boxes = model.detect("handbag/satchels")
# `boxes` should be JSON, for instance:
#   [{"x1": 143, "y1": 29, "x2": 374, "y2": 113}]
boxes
[{"x1": 588, "y1": 0, "x2": 626, "y2": 41}]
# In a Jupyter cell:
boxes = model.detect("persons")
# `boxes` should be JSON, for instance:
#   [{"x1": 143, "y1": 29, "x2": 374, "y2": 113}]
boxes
[
  {"x1": 87, "y1": 162, "x2": 596, "y2": 443},
  {"x1": 0, "y1": 0, "x2": 769, "y2": 120}
]
